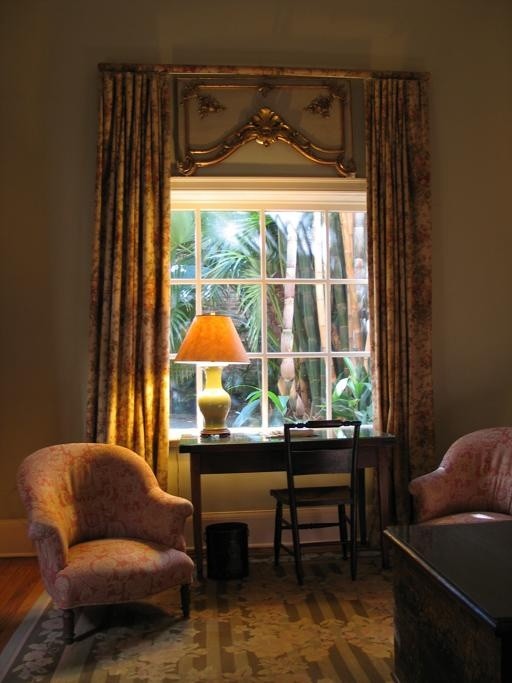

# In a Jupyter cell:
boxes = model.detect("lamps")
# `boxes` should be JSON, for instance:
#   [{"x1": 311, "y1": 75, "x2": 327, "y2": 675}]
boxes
[{"x1": 173, "y1": 312, "x2": 252, "y2": 438}]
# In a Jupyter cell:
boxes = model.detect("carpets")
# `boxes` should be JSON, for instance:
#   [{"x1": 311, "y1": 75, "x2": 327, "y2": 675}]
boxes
[{"x1": 2, "y1": 544, "x2": 400, "y2": 682}]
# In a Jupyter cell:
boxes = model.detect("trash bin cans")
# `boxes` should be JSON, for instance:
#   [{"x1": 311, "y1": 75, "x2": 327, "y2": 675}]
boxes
[{"x1": 205, "y1": 522, "x2": 250, "y2": 580}]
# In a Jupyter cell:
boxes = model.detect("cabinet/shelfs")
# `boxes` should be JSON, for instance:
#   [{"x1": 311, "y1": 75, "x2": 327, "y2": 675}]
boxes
[{"x1": 383, "y1": 520, "x2": 512, "y2": 682}]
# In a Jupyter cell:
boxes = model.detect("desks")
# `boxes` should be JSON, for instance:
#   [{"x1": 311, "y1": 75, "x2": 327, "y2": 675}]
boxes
[{"x1": 178, "y1": 428, "x2": 398, "y2": 581}]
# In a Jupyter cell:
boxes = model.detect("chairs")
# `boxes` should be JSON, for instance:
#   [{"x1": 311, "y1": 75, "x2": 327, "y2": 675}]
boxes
[
  {"x1": 269, "y1": 420, "x2": 362, "y2": 587},
  {"x1": 15, "y1": 443, "x2": 196, "y2": 644},
  {"x1": 408, "y1": 426, "x2": 512, "y2": 525}
]
